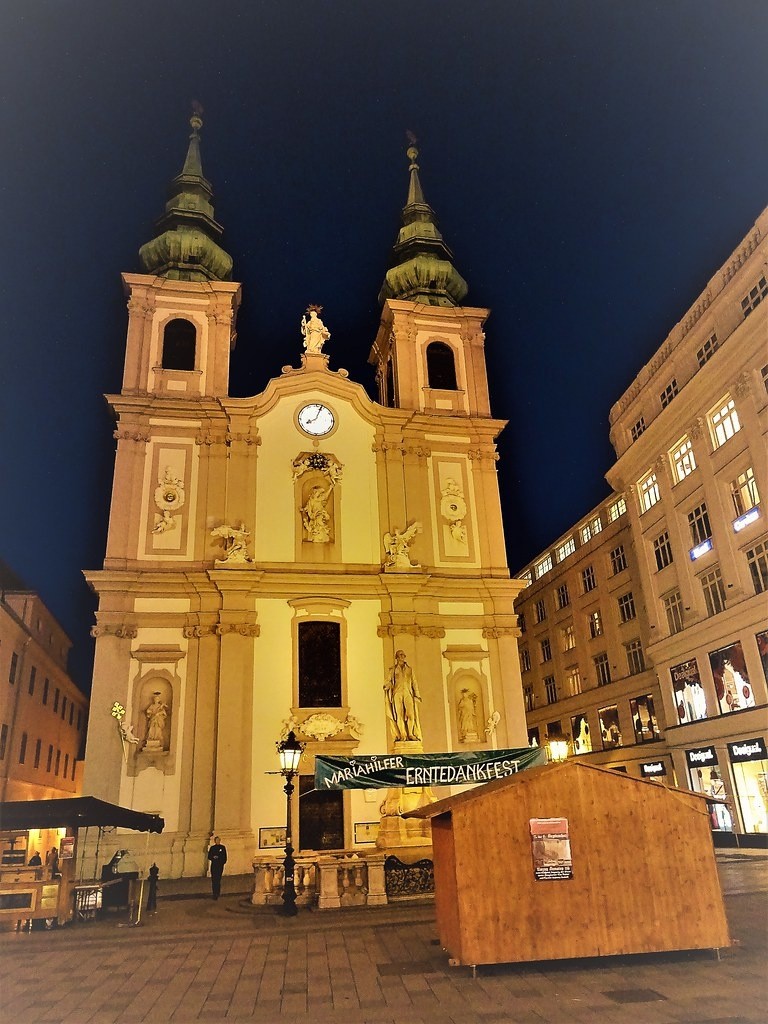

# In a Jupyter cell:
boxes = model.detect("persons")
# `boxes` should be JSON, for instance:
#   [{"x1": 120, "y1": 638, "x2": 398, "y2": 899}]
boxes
[
  {"x1": 151, "y1": 510, "x2": 176, "y2": 535},
  {"x1": 384, "y1": 650, "x2": 423, "y2": 741},
  {"x1": 291, "y1": 459, "x2": 313, "y2": 482},
  {"x1": 118, "y1": 722, "x2": 140, "y2": 745},
  {"x1": 484, "y1": 711, "x2": 501, "y2": 736},
  {"x1": 449, "y1": 521, "x2": 467, "y2": 546},
  {"x1": 299, "y1": 483, "x2": 334, "y2": 543},
  {"x1": 382, "y1": 522, "x2": 423, "y2": 573},
  {"x1": 456, "y1": 687, "x2": 481, "y2": 743},
  {"x1": 140, "y1": 696, "x2": 168, "y2": 752},
  {"x1": 207, "y1": 836, "x2": 227, "y2": 897},
  {"x1": 321, "y1": 459, "x2": 342, "y2": 486},
  {"x1": 210, "y1": 521, "x2": 257, "y2": 564},
  {"x1": 301, "y1": 304, "x2": 331, "y2": 355}
]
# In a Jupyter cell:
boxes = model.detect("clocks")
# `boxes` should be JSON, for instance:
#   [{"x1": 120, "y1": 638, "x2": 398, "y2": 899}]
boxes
[{"x1": 297, "y1": 403, "x2": 335, "y2": 436}]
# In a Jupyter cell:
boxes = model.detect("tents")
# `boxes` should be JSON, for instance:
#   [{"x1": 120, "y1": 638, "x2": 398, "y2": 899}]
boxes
[{"x1": 0, "y1": 795, "x2": 164, "y2": 930}]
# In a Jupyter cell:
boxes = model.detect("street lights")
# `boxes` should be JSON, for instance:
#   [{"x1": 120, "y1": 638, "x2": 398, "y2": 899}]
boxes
[{"x1": 275, "y1": 730, "x2": 308, "y2": 915}]
